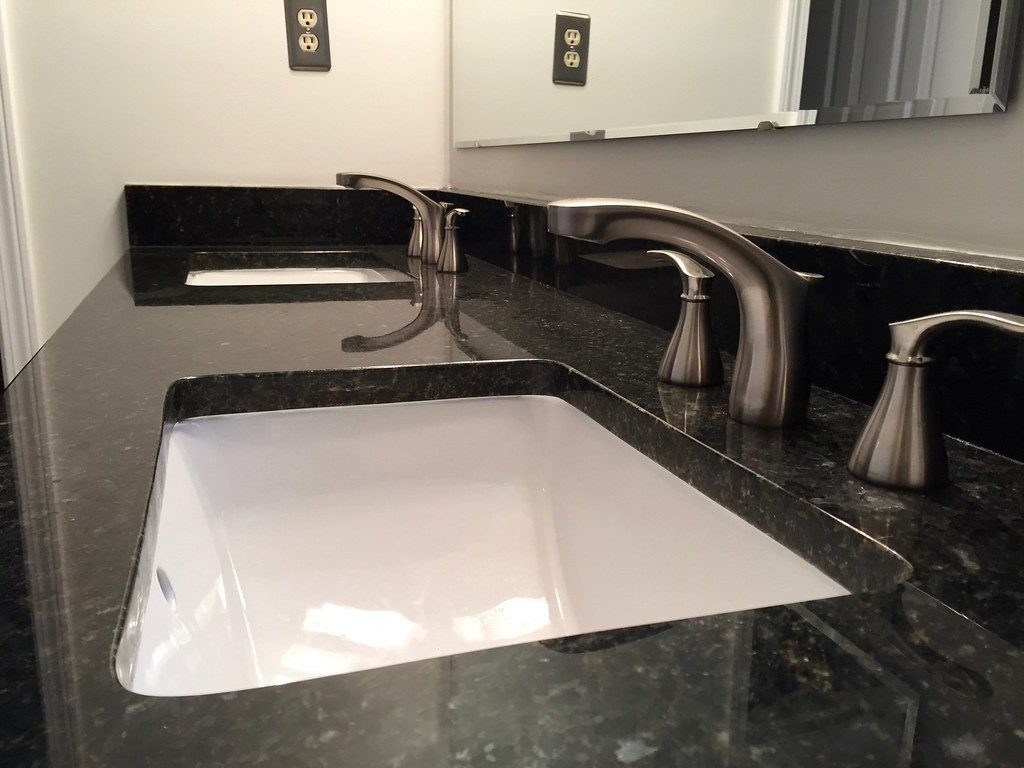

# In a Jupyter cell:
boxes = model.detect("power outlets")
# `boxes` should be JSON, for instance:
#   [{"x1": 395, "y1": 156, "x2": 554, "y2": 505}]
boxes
[
  {"x1": 554, "y1": 11, "x2": 591, "y2": 86},
  {"x1": 283, "y1": 0, "x2": 331, "y2": 71}
]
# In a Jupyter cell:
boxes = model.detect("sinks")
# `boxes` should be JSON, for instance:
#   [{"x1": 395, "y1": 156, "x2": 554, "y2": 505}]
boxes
[
  {"x1": 182, "y1": 249, "x2": 424, "y2": 287},
  {"x1": 109, "y1": 357, "x2": 912, "y2": 701}
]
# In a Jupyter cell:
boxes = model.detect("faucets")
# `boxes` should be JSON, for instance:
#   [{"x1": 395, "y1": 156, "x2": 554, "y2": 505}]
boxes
[
  {"x1": 338, "y1": 269, "x2": 444, "y2": 354},
  {"x1": 336, "y1": 168, "x2": 455, "y2": 264},
  {"x1": 545, "y1": 197, "x2": 824, "y2": 430}
]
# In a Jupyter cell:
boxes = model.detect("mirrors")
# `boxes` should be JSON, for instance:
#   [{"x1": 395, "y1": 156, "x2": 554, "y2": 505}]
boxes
[{"x1": 447, "y1": 0, "x2": 1021, "y2": 151}]
